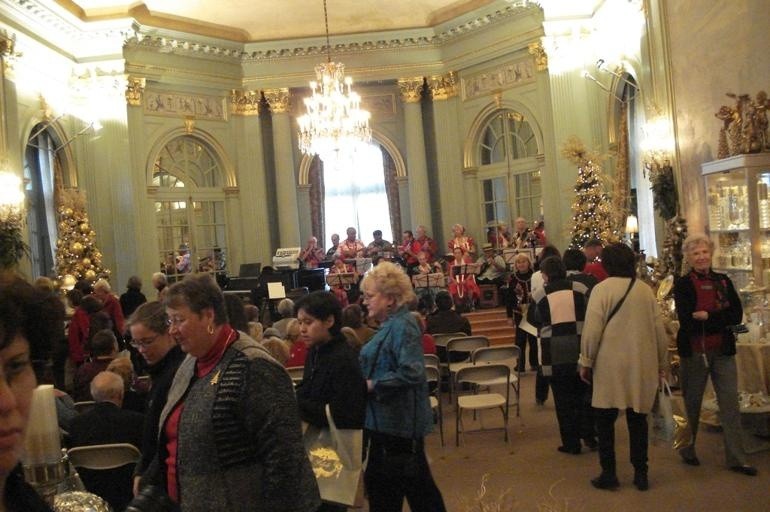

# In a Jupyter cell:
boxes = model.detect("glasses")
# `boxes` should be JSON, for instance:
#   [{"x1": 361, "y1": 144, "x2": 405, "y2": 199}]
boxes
[{"x1": 129, "y1": 331, "x2": 161, "y2": 347}]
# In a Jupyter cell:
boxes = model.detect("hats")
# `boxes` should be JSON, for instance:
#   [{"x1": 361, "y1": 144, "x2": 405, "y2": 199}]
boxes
[{"x1": 482, "y1": 243, "x2": 494, "y2": 251}]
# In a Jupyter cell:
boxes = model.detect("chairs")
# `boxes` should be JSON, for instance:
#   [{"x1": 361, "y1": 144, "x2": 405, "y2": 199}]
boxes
[
  {"x1": 421, "y1": 330, "x2": 526, "y2": 450},
  {"x1": 63, "y1": 444, "x2": 141, "y2": 474},
  {"x1": 128, "y1": 372, "x2": 152, "y2": 391},
  {"x1": 71, "y1": 400, "x2": 96, "y2": 413},
  {"x1": 287, "y1": 364, "x2": 306, "y2": 384}
]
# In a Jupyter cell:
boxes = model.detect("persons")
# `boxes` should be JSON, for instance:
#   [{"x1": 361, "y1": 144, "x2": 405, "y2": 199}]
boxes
[
  {"x1": 302, "y1": 217, "x2": 545, "y2": 311},
  {"x1": 1, "y1": 260, "x2": 444, "y2": 512},
  {"x1": 670, "y1": 234, "x2": 759, "y2": 477},
  {"x1": 507, "y1": 237, "x2": 671, "y2": 491}
]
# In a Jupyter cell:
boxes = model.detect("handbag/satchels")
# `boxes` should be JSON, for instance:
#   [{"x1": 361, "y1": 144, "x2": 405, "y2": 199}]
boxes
[
  {"x1": 300, "y1": 404, "x2": 366, "y2": 509},
  {"x1": 126, "y1": 485, "x2": 180, "y2": 512},
  {"x1": 651, "y1": 378, "x2": 692, "y2": 451}
]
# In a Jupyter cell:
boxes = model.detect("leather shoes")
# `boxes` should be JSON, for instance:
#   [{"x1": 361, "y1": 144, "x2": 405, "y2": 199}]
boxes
[
  {"x1": 634, "y1": 469, "x2": 649, "y2": 490},
  {"x1": 684, "y1": 454, "x2": 698, "y2": 465},
  {"x1": 732, "y1": 464, "x2": 757, "y2": 475},
  {"x1": 590, "y1": 473, "x2": 620, "y2": 490}
]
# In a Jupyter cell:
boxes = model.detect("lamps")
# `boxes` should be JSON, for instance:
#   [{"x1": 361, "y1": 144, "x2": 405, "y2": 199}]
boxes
[
  {"x1": 576, "y1": 58, "x2": 644, "y2": 108},
  {"x1": 624, "y1": 214, "x2": 639, "y2": 243},
  {"x1": 284, "y1": 0, "x2": 374, "y2": 165}
]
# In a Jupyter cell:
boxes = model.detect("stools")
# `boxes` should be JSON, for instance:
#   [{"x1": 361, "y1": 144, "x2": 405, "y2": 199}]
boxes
[{"x1": 477, "y1": 282, "x2": 504, "y2": 309}]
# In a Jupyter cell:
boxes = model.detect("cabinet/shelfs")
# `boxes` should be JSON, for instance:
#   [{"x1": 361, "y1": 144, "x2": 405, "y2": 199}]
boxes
[{"x1": 699, "y1": 154, "x2": 769, "y2": 442}]
